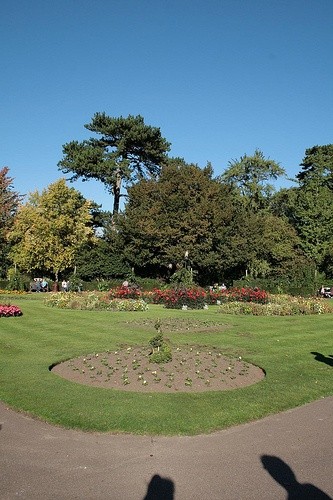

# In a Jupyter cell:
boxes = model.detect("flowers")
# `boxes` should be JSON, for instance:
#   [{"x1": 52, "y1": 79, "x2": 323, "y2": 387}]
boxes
[{"x1": 109, "y1": 281, "x2": 269, "y2": 310}]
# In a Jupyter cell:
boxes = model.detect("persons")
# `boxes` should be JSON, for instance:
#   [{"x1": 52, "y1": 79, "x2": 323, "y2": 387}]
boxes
[
  {"x1": 40, "y1": 279, "x2": 48, "y2": 292},
  {"x1": 35, "y1": 278, "x2": 41, "y2": 292}
]
